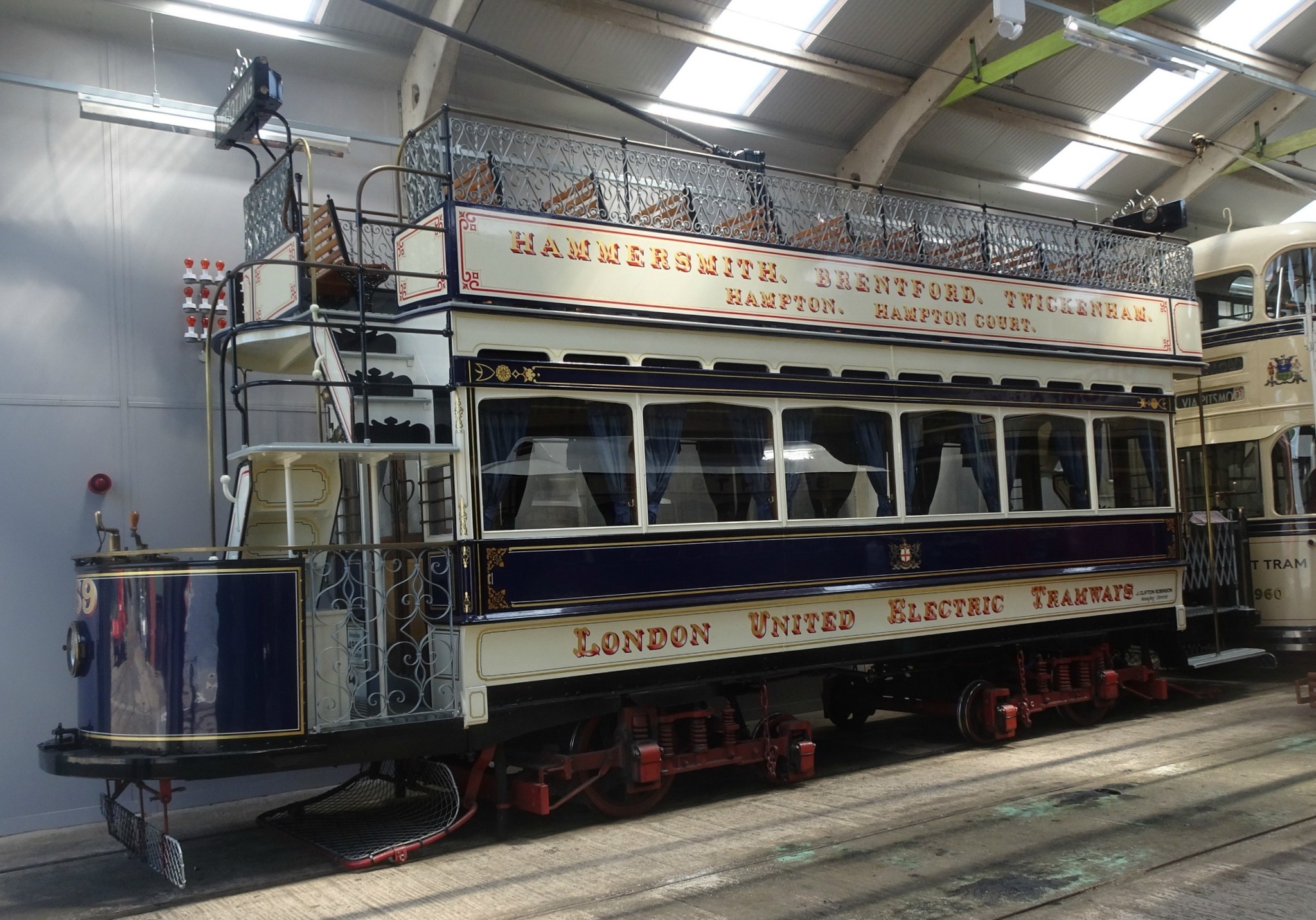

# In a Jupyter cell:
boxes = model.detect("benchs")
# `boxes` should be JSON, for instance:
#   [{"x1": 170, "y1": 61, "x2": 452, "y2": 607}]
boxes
[
  {"x1": 447, "y1": 153, "x2": 1151, "y2": 295},
  {"x1": 297, "y1": 196, "x2": 392, "y2": 307}
]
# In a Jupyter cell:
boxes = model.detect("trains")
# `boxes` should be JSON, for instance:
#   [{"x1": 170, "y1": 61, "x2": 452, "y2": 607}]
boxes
[{"x1": 33, "y1": 45, "x2": 1316, "y2": 889}]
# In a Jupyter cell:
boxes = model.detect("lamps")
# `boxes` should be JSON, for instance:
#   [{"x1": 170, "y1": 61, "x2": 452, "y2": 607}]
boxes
[{"x1": 993, "y1": 0, "x2": 1026, "y2": 41}]
